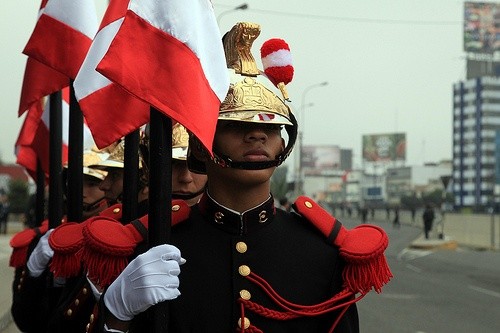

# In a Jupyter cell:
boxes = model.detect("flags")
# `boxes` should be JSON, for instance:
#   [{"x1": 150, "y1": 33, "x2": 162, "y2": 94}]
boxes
[
  {"x1": 71, "y1": 1, "x2": 149, "y2": 150},
  {"x1": 14, "y1": 84, "x2": 93, "y2": 187},
  {"x1": 18, "y1": 0, "x2": 70, "y2": 116},
  {"x1": 94, "y1": 0, "x2": 231, "y2": 158},
  {"x1": 21, "y1": 0, "x2": 111, "y2": 79}
]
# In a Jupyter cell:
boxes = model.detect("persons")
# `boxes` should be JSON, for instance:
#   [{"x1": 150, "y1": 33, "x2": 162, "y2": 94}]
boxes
[
  {"x1": 53, "y1": 121, "x2": 210, "y2": 333},
  {"x1": 89, "y1": 70, "x2": 364, "y2": 333},
  {"x1": 315, "y1": 198, "x2": 446, "y2": 241},
  {"x1": 0, "y1": 193, "x2": 10, "y2": 234},
  {"x1": 279, "y1": 196, "x2": 302, "y2": 216},
  {"x1": 9, "y1": 123, "x2": 148, "y2": 333}
]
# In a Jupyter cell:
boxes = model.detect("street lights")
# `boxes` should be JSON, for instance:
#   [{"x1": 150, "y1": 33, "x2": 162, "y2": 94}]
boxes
[
  {"x1": 294, "y1": 81, "x2": 328, "y2": 193},
  {"x1": 217, "y1": 3, "x2": 248, "y2": 28}
]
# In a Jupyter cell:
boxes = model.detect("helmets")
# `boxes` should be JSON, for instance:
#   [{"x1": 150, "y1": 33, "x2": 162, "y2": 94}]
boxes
[
  {"x1": 87, "y1": 139, "x2": 143, "y2": 172},
  {"x1": 171, "y1": 122, "x2": 190, "y2": 160},
  {"x1": 186, "y1": 21, "x2": 293, "y2": 174},
  {"x1": 63, "y1": 147, "x2": 110, "y2": 180}
]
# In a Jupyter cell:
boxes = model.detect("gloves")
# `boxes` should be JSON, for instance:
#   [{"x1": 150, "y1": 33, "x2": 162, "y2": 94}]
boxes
[
  {"x1": 27, "y1": 229, "x2": 54, "y2": 277},
  {"x1": 104, "y1": 244, "x2": 187, "y2": 321}
]
